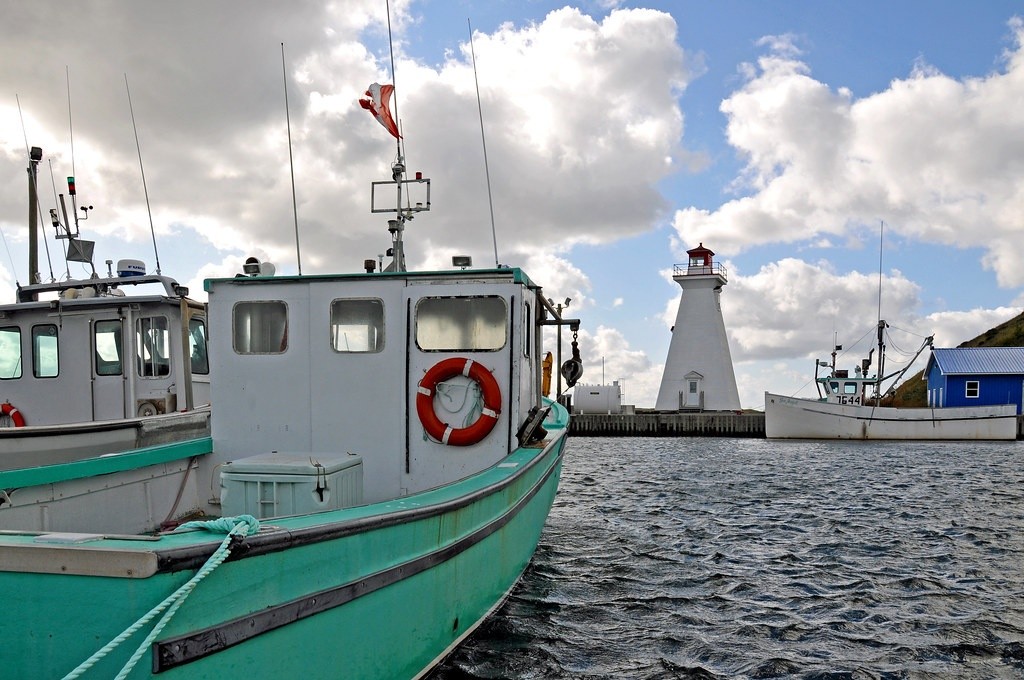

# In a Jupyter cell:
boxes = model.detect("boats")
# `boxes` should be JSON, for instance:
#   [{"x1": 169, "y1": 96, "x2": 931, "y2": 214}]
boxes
[
  {"x1": 764, "y1": 319, "x2": 1018, "y2": 442},
  {"x1": 0, "y1": 0, "x2": 585, "y2": 680}
]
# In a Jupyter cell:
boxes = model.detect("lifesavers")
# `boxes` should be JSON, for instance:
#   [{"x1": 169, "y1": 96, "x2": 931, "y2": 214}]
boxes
[
  {"x1": 415, "y1": 357, "x2": 502, "y2": 446},
  {"x1": 0, "y1": 404, "x2": 25, "y2": 427}
]
